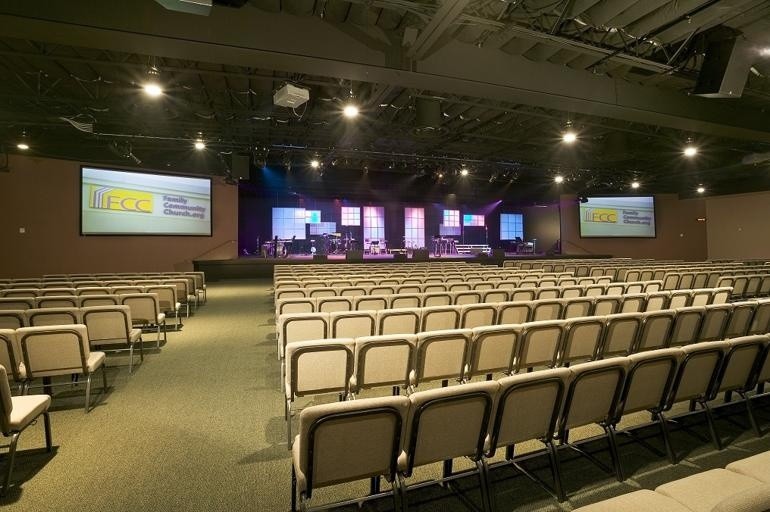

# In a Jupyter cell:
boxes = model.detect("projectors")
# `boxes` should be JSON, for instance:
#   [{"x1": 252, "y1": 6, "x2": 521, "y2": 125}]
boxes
[{"x1": 273, "y1": 84, "x2": 311, "y2": 110}]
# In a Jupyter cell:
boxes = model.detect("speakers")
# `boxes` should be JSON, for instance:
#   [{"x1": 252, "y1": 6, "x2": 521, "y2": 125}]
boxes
[
  {"x1": 232, "y1": 155, "x2": 250, "y2": 179},
  {"x1": 691, "y1": 36, "x2": 754, "y2": 97}
]
